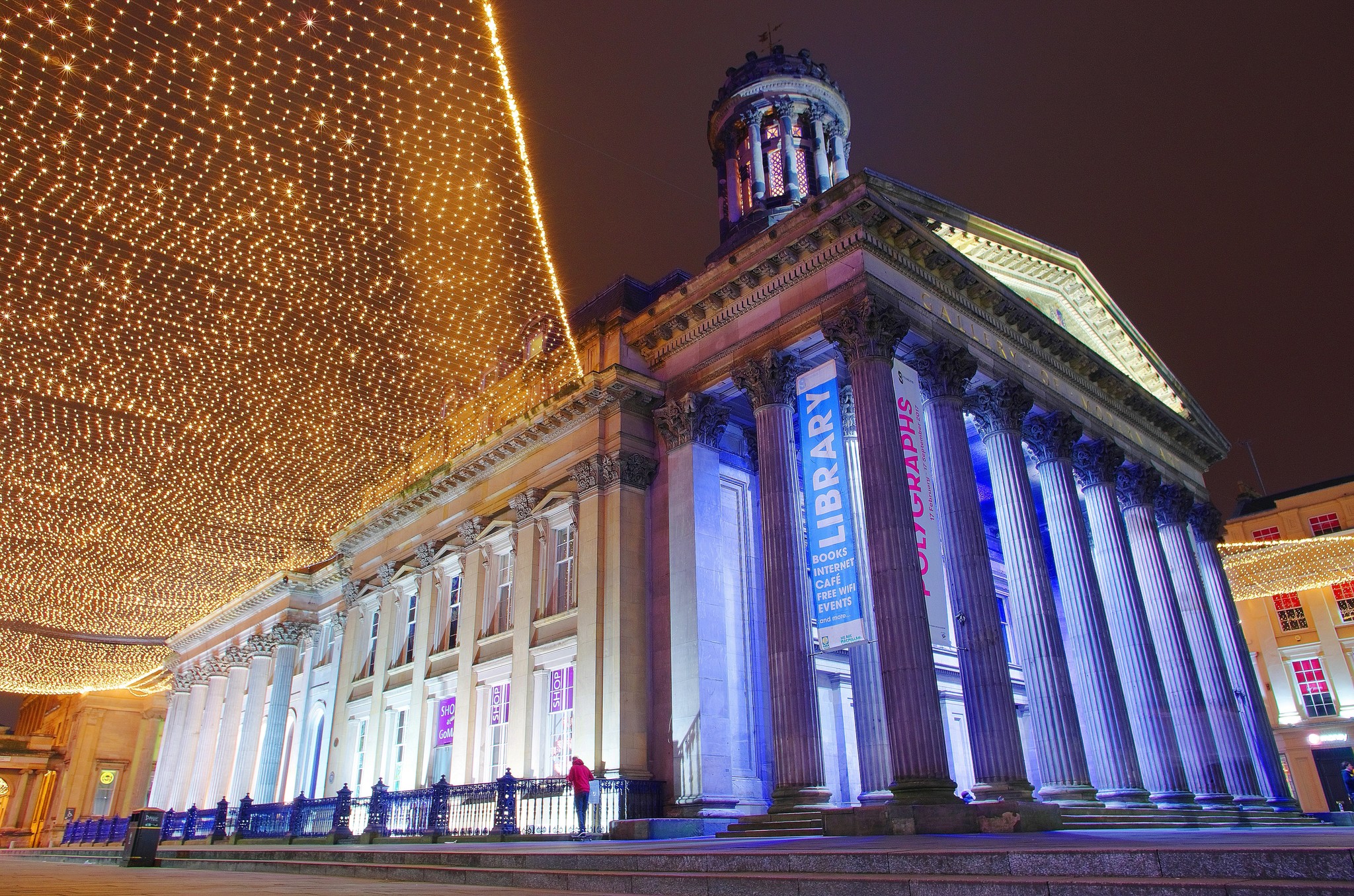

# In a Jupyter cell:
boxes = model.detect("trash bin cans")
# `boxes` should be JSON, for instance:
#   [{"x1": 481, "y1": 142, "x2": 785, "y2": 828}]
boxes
[{"x1": 119, "y1": 807, "x2": 167, "y2": 866}]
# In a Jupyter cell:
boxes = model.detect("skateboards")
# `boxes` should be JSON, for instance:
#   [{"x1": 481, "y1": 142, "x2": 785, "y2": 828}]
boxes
[{"x1": 571, "y1": 834, "x2": 592, "y2": 842}]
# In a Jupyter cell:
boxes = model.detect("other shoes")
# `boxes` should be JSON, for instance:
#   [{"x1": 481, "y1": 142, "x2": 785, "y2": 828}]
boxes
[{"x1": 578, "y1": 830, "x2": 586, "y2": 836}]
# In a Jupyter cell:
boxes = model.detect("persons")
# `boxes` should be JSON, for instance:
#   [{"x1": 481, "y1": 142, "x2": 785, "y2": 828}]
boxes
[
  {"x1": 961, "y1": 790, "x2": 974, "y2": 804},
  {"x1": 1341, "y1": 761, "x2": 1354, "y2": 807},
  {"x1": 565, "y1": 756, "x2": 594, "y2": 838}
]
